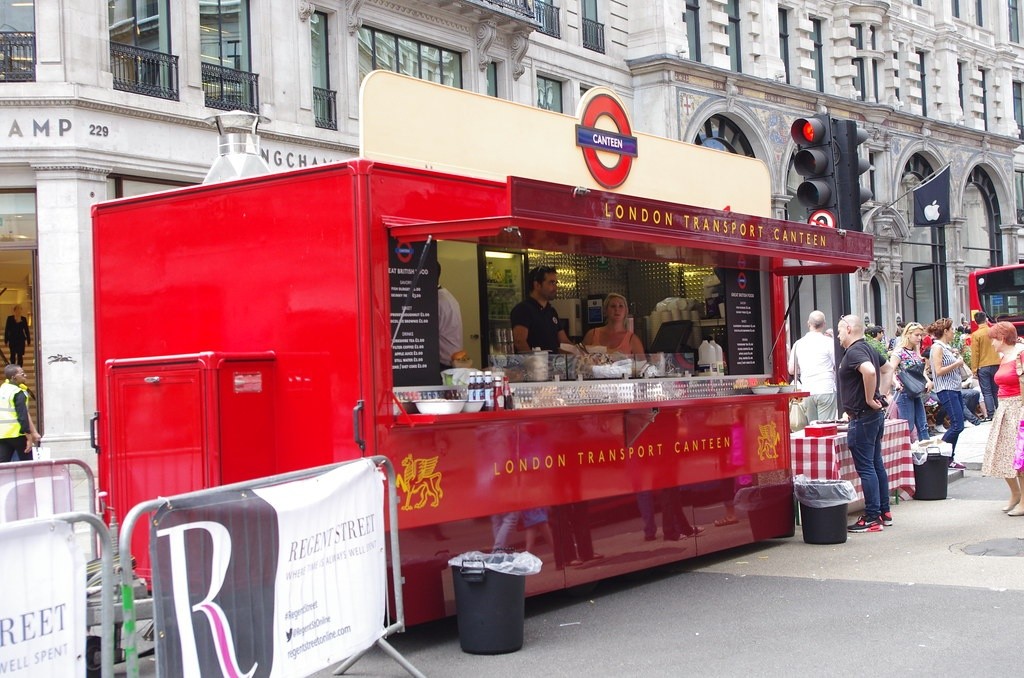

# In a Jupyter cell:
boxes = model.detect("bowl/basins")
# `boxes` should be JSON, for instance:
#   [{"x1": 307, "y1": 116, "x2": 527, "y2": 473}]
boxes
[
  {"x1": 463, "y1": 399, "x2": 485, "y2": 413},
  {"x1": 649, "y1": 298, "x2": 702, "y2": 356},
  {"x1": 412, "y1": 399, "x2": 466, "y2": 415}
]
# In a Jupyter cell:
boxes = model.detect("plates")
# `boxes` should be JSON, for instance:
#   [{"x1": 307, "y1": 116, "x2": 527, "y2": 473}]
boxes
[
  {"x1": 750, "y1": 385, "x2": 780, "y2": 395},
  {"x1": 779, "y1": 385, "x2": 796, "y2": 392}
]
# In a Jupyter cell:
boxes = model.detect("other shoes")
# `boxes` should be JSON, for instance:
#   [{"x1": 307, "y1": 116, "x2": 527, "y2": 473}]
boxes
[
  {"x1": 556, "y1": 553, "x2": 604, "y2": 565},
  {"x1": 1002, "y1": 493, "x2": 1021, "y2": 512},
  {"x1": 677, "y1": 525, "x2": 705, "y2": 540},
  {"x1": 1008, "y1": 503, "x2": 1024, "y2": 516}
]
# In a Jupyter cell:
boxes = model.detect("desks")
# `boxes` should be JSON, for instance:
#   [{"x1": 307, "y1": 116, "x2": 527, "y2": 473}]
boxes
[{"x1": 789, "y1": 418, "x2": 916, "y2": 528}]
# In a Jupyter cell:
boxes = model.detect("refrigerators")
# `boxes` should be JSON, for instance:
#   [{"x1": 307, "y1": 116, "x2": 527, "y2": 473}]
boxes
[{"x1": 435, "y1": 238, "x2": 528, "y2": 375}]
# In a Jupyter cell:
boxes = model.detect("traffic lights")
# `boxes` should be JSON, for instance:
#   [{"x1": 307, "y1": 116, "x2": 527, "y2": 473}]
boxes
[
  {"x1": 790, "y1": 114, "x2": 839, "y2": 229},
  {"x1": 832, "y1": 117, "x2": 874, "y2": 232}
]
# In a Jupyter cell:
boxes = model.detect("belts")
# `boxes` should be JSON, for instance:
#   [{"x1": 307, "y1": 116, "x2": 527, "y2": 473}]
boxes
[{"x1": 848, "y1": 408, "x2": 879, "y2": 421}]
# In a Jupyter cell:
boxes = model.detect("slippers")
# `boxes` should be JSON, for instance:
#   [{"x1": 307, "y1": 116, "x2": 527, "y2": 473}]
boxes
[{"x1": 714, "y1": 516, "x2": 739, "y2": 526}]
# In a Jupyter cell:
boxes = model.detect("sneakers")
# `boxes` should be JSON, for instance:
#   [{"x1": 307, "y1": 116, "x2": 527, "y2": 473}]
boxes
[
  {"x1": 947, "y1": 457, "x2": 966, "y2": 470},
  {"x1": 877, "y1": 511, "x2": 892, "y2": 525},
  {"x1": 847, "y1": 514, "x2": 884, "y2": 533},
  {"x1": 963, "y1": 419, "x2": 975, "y2": 427},
  {"x1": 934, "y1": 423, "x2": 947, "y2": 433}
]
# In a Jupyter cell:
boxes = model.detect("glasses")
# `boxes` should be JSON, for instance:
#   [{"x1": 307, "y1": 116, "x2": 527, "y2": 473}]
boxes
[
  {"x1": 942, "y1": 317, "x2": 947, "y2": 326},
  {"x1": 905, "y1": 323, "x2": 918, "y2": 335},
  {"x1": 839, "y1": 315, "x2": 850, "y2": 326},
  {"x1": 533, "y1": 265, "x2": 551, "y2": 280},
  {"x1": 16, "y1": 372, "x2": 24, "y2": 376}
]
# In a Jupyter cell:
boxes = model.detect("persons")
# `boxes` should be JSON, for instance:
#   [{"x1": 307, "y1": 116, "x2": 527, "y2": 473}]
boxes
[
  {"x1": 871, "y1": 326, "x2": 884, "y2": 342},
  {"x1": 4, "y1": 304, "x2": 30, "y2": 368},
  {"x1": 823, "y1": 329, "x2": 834, "y2": 339},
  {"x1": 890, "y1": 322, "x2": 932, "y2": 442},
  {"x1": 895, "y1": 322, "x2": 905, "y2": 337},
  {"x1": 510, "y1": 266, "x2": 574, "y2": 355},
  {"x1": 0, "y1": 364, "x2": 40, "y2": 463},
  {"x1": 971, "y1": 312, "x2": 1003, "y2": 422},
  {"x1": 582, "y1": 293, "x2": 646, "y2": 361},
  {"x1": 837, "y1": 314, "x2": 894, "y2": 533},
  {"x1": 982, "y1": 321, "x2": 1024, "y2": 516},
  {"x1": 478, "y1": 406, "x2": 748, "y2": 571},
  {"x1": 922, "y1": 318, "x2": 991, "y2": 470},
  {"x1": 437, "y1": 260, "x2": 463, "y2": 385},
  {"x1": 788, "y1": 310, "x2": 837, "y2": 425}
]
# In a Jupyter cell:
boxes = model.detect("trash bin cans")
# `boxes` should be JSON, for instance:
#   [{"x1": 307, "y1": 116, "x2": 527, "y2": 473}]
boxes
[
  {"x1": 912, "y1": 446, "x2": 948, "y2": 500},
  {"x1": 452, "y1": 547, "x2": 525, "y2": 655},
  {"x1": 796, "y1": 480, "x2": 848, "y2": 544},
  {"x1": 386, "y1": 549, "x2": 449, "y2": 627},
  {"x1": 745, "y1": 482, "x2": 795, "y2": 540}
]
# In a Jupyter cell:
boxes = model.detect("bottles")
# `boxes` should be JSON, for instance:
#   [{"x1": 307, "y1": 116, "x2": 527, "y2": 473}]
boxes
[
  {"x1": 504, "y1": 268, "x2": 512, "y2": 283},
  {"x1": 468, "y1": 371, "x2": 514, "y2": 412},
  {"x1": 697, "y1": 336, "x2": 724, "y2": 377},
  {"x1": 488, "y1": 261, "x2": 494, "y2": 281}
]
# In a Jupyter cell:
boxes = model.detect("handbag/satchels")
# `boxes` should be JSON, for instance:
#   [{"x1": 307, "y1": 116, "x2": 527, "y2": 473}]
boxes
[
  {"x1": 788, "y1": 400, "x2": 808, "y2": 432},
  {"x1": 1016, "y1": 352, "x2": 1024, "y2": 404},
  {"x1": 736, "y1": 466, "x2": 753, "y2": 486},
  {"x1": 957, "y1": 358, "x2": 972, "y2": 378},
  {"x1": 1014, "y1": 419, "x2": 1024, "y2": 472},
  {"x1": 896, "y1": 347, "x2": 928, "y2": 398}
]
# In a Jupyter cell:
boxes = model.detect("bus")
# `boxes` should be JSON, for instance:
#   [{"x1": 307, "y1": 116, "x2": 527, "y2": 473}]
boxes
[{"x1": 968, "y1": 259, "x2": 1024, "y2": 339}]
